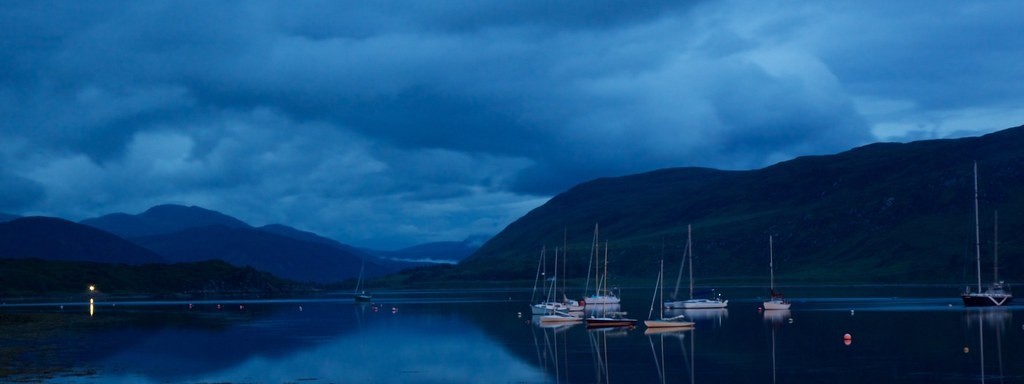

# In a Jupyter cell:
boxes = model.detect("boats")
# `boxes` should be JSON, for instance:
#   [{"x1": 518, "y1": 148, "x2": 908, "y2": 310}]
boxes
[{"x1": 663, "y1": 310, "x2": 728, "y2": 319}]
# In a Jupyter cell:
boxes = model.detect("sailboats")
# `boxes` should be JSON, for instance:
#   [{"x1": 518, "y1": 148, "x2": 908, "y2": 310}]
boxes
[
  {"x1": 664, "y1": 223, "x2": 729, "y2": 309},
  {"x1": 354, "y1": 257, "x2": 372, "y2": 302},
  {"x1": 643, "y1": 259, "x2": 697, "y2": 328},
  {"x1": 644, "y1": 327, "x2": 696, "y2": 384},
  {"x1": 531, "y1": 316, "x2": 584, "y2": 384},
  {"x1": 586, "y1": 326, "x2": 642, "y2": 384},
  {"x1": 959, "y1": 311, "x2": 1013, "y2": 384},
  {"x1": 763, "y1": 235, "x2": 792, "y2": 310},
  {"x1": 764, "y1": 310, "x2": 792, "y2": 384},
  {"x1": 961, "y1": 160, "x2": 1015, "y2": 307},
  {"x1": 529, "y1": 223, "x2": 638, "y2": 331}
]
